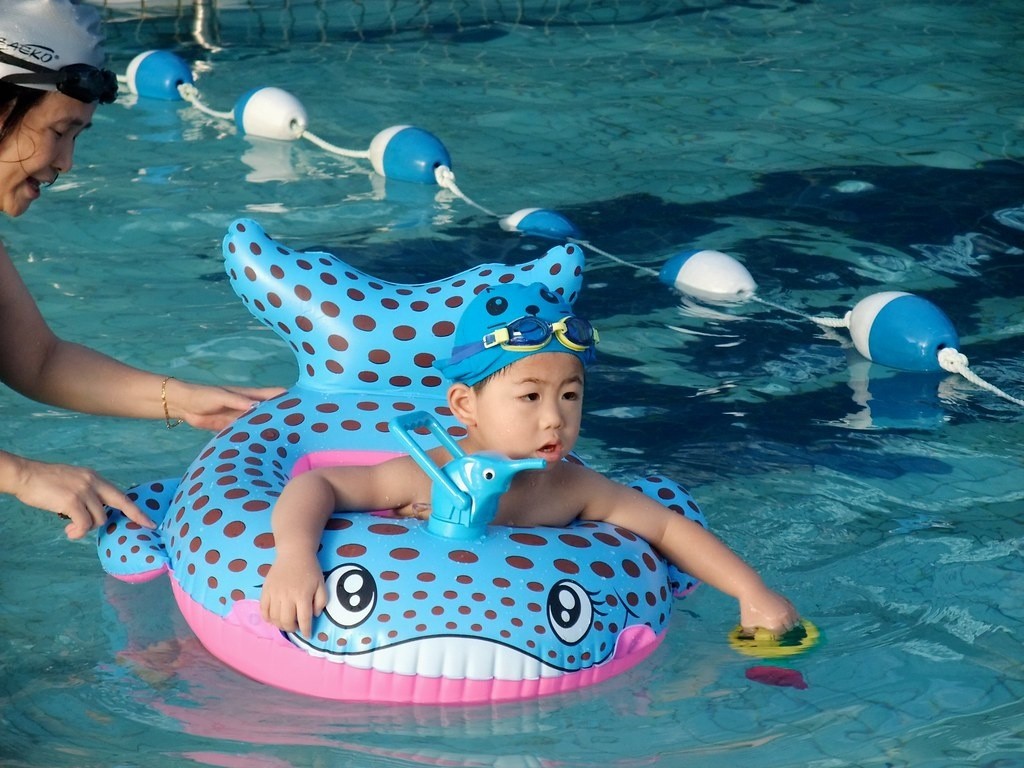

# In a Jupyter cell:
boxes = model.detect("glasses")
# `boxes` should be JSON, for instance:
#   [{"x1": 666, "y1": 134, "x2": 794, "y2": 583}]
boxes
[
  {"x1": 1, "y1": 63, "x2": 118, "y2": 105},
  {"x1": 432, "y1": 316, "x2": 599, "y2": 370}
]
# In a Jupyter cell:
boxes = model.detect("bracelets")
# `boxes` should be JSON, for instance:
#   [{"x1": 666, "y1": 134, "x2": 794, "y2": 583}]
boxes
[{"x1": 162, "y1": 376, "x2": 183, "y2": 429}]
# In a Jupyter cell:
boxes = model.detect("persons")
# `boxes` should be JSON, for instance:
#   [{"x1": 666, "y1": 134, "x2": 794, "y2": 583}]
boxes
[
  {"x1": 259, "y1": 282, "x2": 801, "y2": 639},
  {"x1": 0, "y1": 1, "x2": 286, "y2": 541}
]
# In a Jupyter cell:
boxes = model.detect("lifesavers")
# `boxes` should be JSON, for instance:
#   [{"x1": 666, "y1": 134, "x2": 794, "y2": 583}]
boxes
[{"x1": 98, "y1": 219, "x2": 708, "y2": 707}]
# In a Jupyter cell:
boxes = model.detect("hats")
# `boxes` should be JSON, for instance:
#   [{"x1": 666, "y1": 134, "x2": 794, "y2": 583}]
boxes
[
  {"x1": 1, "y1": 1, "x2": 108, "y2": 92},
  {"x1": 443, "y1": 282, "x2": 585, "y2": 386}
]
